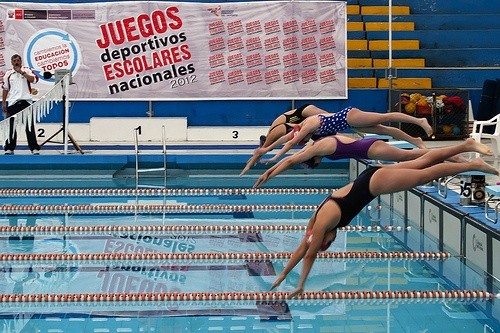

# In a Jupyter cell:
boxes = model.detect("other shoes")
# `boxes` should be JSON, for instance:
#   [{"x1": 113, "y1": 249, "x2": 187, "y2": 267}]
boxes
[
  {"x1": 5, "y1": 150, "x2": 14, "y2": 154},
  {"x1": 33, "y1": 149, "x2": 39, "y2": 155}
]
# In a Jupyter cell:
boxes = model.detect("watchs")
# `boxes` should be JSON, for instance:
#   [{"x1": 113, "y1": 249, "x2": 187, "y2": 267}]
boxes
[{"x1": 22, "y1": 71, "x2": 26, "y2": 75}]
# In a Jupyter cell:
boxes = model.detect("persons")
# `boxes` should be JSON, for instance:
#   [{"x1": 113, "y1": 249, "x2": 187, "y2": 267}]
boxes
[
  {"x1": 2, "y1": 54, "x2": 41, "y2": 155},
  {"x1": 240, "y1": 122, "x2": 298, "y2": 176},
  {"x1": 256, "y1": 107, "x2": 434, "y2": 166},
  {"x1": 254, "y1": 135, "x2": 471, "y2": 188},
  {"x1": 269, "y1": 138, "x2": 500, "y2": 298},
  {"x1": 266, "y1": 104, "x2": 329, "y2": 136}
]
